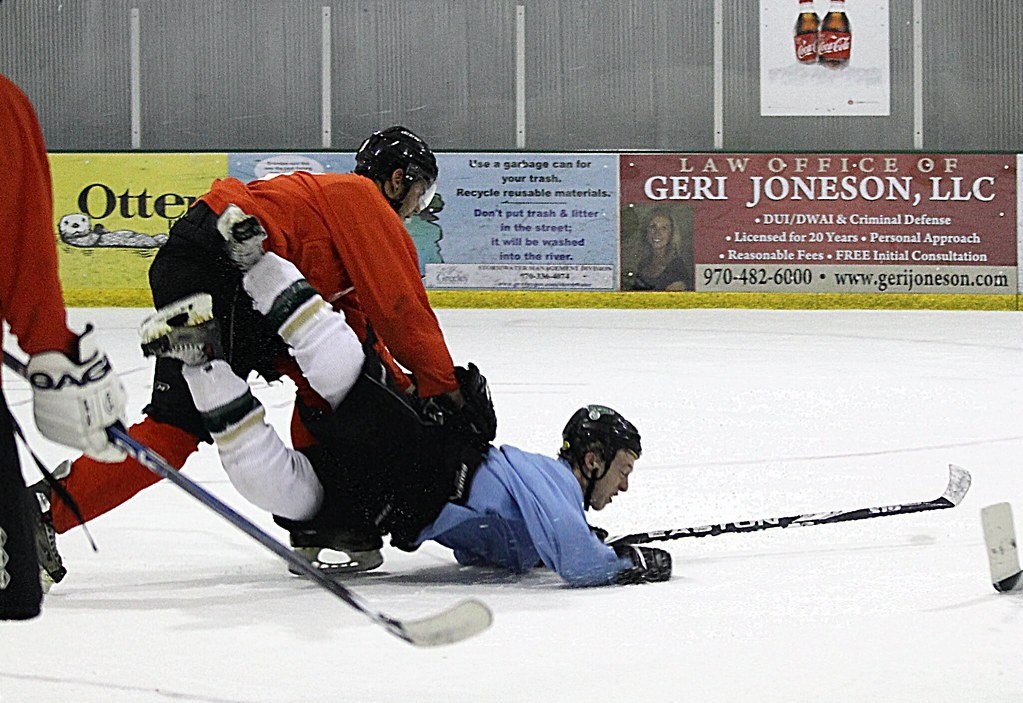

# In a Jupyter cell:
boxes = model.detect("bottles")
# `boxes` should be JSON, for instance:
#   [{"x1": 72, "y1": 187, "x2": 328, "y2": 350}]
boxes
[
  {"x1": 792, "y1": 0, "x2": 821, "y2": 65},
  {"x1": 818, "y1": 0, "x2": 852, "y2": 70}
]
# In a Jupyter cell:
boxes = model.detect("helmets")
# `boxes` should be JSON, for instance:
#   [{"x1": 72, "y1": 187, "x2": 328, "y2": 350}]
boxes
[
  {"x1": 563, "y1": 402, "x2": 641, "y2": 481},
  {"x1": 355, "y1": 125, "x2": 441, "y2": 213}
]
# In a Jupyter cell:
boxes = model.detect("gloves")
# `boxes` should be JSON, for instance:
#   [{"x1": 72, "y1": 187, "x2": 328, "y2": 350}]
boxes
[
  {"x1": 456, "y1": 363, "x2": 497, "y2": 443},
  {"x1": 608, "y1": 540, "x2": 671, "y2": 586},
  {"x1": 29, "y1": 321, "x2": 132, "y2": 461}
]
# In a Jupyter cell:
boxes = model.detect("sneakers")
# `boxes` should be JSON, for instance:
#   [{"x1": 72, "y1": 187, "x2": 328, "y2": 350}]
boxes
[
  {"x1": 25, "y1": 462, "x2": 71, "y2": 597},
  {"x1": 287, "y1": 530, "x2": 383, "y2": 576},
  {"x1": 141, "y1": 293, "x2": 223, "y2": 368},
  {"x1": 216, "y1": 205, "x2": 269, "y2": 272}
]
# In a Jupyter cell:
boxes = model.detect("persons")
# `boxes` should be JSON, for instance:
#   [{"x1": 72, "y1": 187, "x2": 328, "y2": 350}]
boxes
[
  {"x1": 0, "y1": 75, "x2": 131, "y2": 627},
  {"x1": 135, "y1": 203, "x2": 676, "y2": 591},
  {"x1": 29, "y1": 125, "x2": 499, "y2": 586}
]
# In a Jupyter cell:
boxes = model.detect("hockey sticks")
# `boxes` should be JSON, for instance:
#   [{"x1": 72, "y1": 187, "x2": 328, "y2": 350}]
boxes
[
  {"x1": 1, "y1": 348, "x2": 492, "y2": 646},
  {"x1": 608, "y1": 464, "x2": 972, "y2": 555},
  {"x1": 979, "y1": 501, "x2": 1023, "y2": 597}
]
